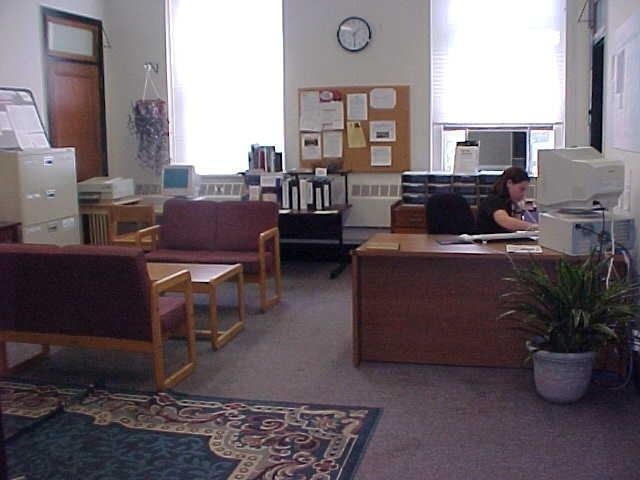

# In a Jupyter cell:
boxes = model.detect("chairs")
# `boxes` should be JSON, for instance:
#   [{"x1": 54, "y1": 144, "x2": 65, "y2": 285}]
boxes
[{"x1": 108, "y1": 204, "x2": 159, "y2": 252}]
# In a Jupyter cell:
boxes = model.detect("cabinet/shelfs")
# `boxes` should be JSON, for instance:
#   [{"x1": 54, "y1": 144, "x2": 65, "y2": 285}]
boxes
[
  {"x1": 1, "y1": 146, "x2": 81, "y2": 246},
  {"x1": 391, "y1": 200, "x2": 428, "y2": 234}
]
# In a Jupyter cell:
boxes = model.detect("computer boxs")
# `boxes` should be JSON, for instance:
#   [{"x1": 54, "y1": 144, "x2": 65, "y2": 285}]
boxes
[{"x1": 539, "y1": 213, "x2": 635, "y2": 256}]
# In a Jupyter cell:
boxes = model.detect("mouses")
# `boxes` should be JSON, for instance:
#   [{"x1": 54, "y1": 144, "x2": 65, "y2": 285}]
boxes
[{"x1": 459, "y1": 234, "x2": 472, "y2": 241}]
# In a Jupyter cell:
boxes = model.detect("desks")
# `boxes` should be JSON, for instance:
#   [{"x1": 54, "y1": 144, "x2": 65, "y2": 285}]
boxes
[
  {"x1": 350, "y1": 233, "x2": 629, "y2": 367},
  {"x1": 279, "y1": 204, "x2": 352, "y2": 261}
]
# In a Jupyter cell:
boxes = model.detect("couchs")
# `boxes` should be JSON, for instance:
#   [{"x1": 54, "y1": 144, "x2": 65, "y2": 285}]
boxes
[
  {"x1": 0, "y1": 244, "x2": 198, "y2": 392},
  {"x1": 135, "y1": 198, "x2": 282, "y2": 312}
]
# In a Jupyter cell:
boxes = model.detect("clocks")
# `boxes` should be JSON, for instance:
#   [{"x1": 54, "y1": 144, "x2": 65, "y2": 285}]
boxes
[{"x1": 336, "y1": 16, "x2": 371, "y2": 52}]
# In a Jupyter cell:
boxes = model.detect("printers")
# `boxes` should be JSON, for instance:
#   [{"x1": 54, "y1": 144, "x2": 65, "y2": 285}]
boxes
[{"x1": 78, "y1": 176, "x2": 134, "y2": 203}]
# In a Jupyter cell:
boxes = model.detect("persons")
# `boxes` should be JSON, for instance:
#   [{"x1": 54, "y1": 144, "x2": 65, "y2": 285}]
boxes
[{"x1": 475, "y1": 167, "x2": 539, "y2": 234}]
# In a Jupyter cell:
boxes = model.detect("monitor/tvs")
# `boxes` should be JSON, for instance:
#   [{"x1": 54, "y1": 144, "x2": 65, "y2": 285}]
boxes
[
  {"x1": 535, "y1": 147, "x2": 625, "y2": 217},
  {"x1": 161, "y1": 165, "x2": 200, "y2": 196}
]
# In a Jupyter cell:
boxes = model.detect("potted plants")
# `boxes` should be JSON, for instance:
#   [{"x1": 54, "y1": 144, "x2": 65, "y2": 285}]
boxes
[{"x1": 494, "y1": 242, "x2": 639, "y2": 403}]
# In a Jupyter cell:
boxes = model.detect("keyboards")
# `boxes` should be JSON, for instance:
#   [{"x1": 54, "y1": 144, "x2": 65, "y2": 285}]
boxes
[{"x1": 470, "y1": 232, "x2": 540, "y2": 243}]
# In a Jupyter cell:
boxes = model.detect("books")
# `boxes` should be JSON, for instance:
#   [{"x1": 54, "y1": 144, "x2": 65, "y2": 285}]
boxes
[{"x1": 244, "y1": 143, "x2": 331, "y2": 210}]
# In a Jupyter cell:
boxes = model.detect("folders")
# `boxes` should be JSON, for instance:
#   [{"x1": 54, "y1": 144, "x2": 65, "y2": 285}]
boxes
[{"x1": 282, "y1": 177, "x2": 335, "y2": 210}]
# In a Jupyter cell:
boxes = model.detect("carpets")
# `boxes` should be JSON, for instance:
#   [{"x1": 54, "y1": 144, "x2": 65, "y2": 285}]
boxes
[{"x1": 0, "y1": 375, "x2": 386, "y2": 480}]
